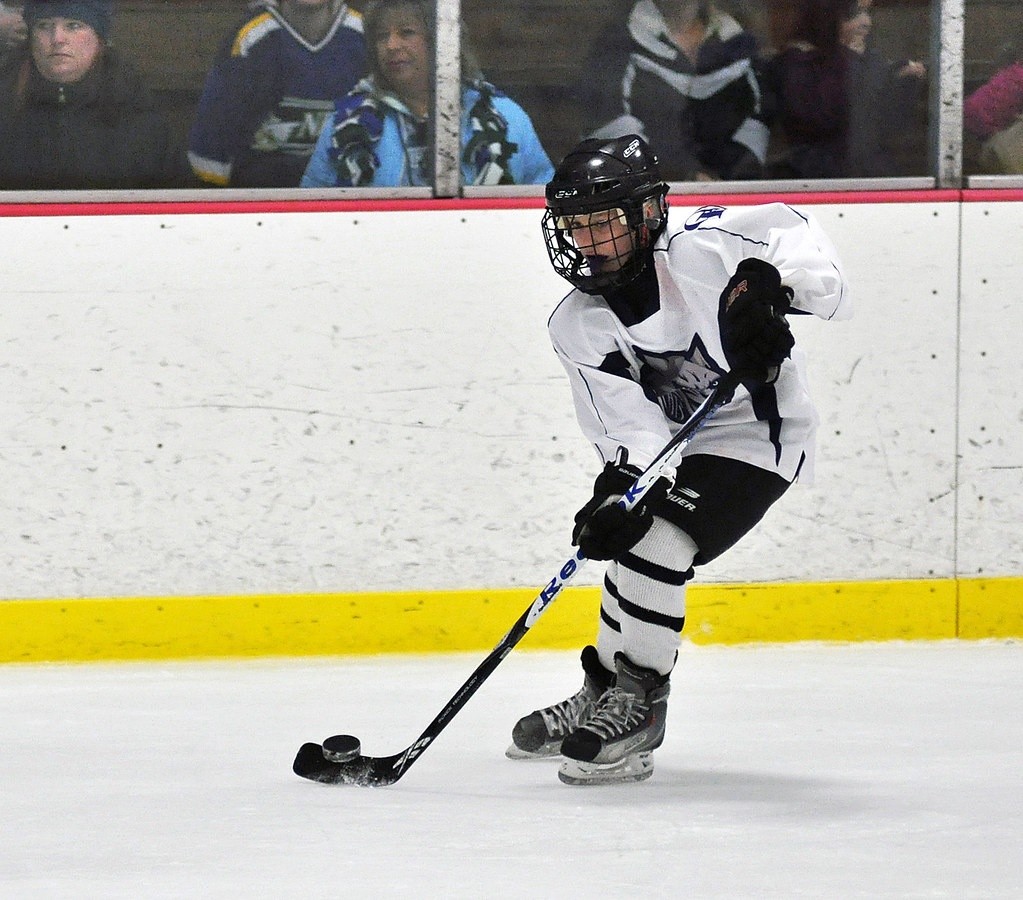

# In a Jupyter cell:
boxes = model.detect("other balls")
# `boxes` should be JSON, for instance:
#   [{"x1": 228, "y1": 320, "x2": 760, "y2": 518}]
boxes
[{"x1": 321, "y1": 734, "x2": 362, "y2": 764}]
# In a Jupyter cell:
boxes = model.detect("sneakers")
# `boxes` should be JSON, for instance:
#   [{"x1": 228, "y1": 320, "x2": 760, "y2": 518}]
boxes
[
  {"x1": 556, "y1": 652, "x2": 680, "y2": 786},
  {"x1": 506, "y1": 646, "x2": 620, "y2": 761}
]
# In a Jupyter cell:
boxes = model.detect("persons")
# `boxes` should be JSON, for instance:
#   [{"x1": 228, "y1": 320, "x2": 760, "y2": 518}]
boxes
[
  {"x1": 186, "y1": 0, "x2": 366, "y2": 190},
  {"x1": 595, "y1": 0, "x2": 771, "y2": 182},
  {"x1": 297, "y1": 0, "x2": 555, "y2": 187},
  {"x1": 0, "y1": 0, "x2": 179, "y2": 190},
  {"x1": 505, "y1": 134, "x2": 855, "y2": 786},
  {"x1": 773, "y1": 0, "x2": 1023, "y2": 178}
]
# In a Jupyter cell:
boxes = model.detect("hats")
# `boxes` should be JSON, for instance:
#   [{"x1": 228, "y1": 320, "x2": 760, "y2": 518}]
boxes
[{"x1": 22, "y1": 1, "x2": 108, "y2": 37}]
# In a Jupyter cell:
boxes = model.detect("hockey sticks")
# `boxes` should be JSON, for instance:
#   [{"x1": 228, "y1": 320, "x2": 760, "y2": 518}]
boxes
[{"x1": 291, "y1": 368, "x2": 740, "y2": 788}]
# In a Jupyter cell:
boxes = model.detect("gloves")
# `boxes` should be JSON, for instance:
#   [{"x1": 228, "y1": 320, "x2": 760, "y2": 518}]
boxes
[
  {"x1": 717, "y1": 257, "x2": 797, "y2": 392},
  {"x1": 570, "y1": 461, "x2": 656, "y2": 561}
]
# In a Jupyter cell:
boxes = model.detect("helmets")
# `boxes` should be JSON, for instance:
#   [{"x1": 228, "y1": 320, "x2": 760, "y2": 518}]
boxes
[{"x1": 540, "y1": 135, "x2": 671, "y2": 295}]
